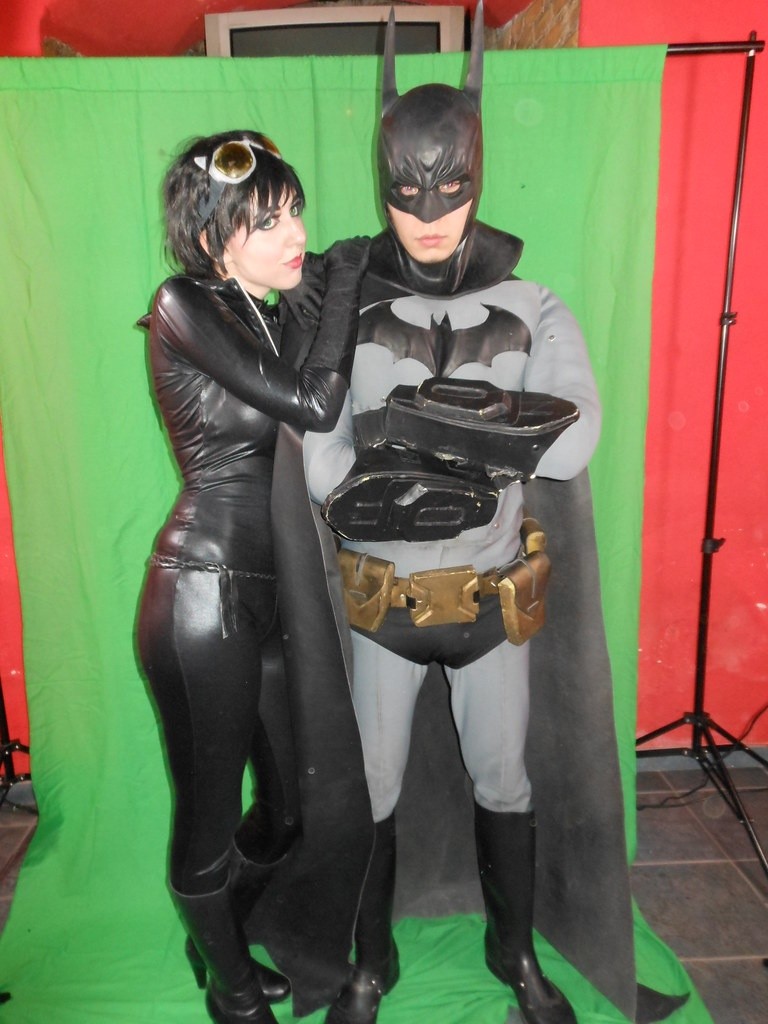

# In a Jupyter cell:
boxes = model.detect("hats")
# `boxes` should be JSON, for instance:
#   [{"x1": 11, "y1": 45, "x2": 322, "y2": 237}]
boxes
[{"x1": 372, "y1": 0, "x2": 484, "y2": 246}]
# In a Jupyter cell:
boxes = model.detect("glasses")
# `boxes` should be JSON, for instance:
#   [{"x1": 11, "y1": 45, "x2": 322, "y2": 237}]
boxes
[{"x1": 190, "y1": 138, "x2": 283, "y2": 239}]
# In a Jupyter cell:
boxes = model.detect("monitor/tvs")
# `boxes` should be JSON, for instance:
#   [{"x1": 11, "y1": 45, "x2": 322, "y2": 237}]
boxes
[{"x1": 204, "y1": 3, "x2": 464, "y2": 59}]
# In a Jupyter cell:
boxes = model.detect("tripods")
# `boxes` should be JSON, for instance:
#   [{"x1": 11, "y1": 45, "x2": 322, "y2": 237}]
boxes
[{"x1": 632, "y1": 31, "x2": 766, "y2": 876}]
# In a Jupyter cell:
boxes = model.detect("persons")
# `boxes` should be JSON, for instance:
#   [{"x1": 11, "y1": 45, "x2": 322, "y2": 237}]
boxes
[
  {"x1": 130, "y1": 126, "x2": 371, "y2": 1024},
  {"x1": 301, "y1": 83, "x2": 601, "y2": 1023}
]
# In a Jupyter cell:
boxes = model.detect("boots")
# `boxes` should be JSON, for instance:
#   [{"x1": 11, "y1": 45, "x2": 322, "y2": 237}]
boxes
[
  {"x1": 471, "y1": 797, "x2": 576, "y2": 1024},
  {"x1": 186, "y1": 834, "x2": 291, "y2": 1005},
  {"x1": 164, "y1": 864, "x2": 279, "y2": 1024},
  {"x1": 324, "y1": 812, "x2": 401, "y2": 1024}
]
